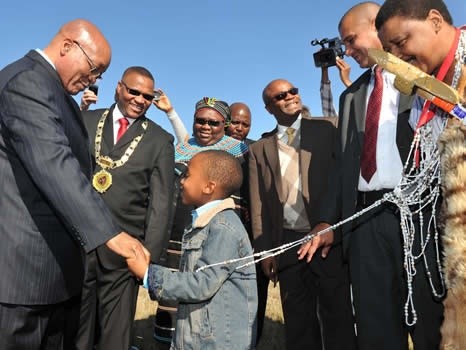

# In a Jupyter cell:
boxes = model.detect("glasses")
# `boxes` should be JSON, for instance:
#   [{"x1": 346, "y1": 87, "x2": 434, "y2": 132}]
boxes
[
  {"x1": 122, "y1": 81, "x2": 155, "y2": 100},
  {"x1": 73, "y1": 41, "x2": 103, "y2": 80},
  {"x1": 271, "y1": 88, "x2": 298, "y2": 103},
  {"x1": 196, "y1": 118, "x2": 220, "y2": 126}
]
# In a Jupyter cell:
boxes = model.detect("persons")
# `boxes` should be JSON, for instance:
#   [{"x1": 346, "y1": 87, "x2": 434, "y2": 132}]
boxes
[
  {"x1": 151, "y1": 97, "x2": 273, "y2": 350},
  {"x1": 127, "y1": 149, "x2": 258, "y2": 350},
  {"x1": 297, "y1": 1, "x2": 448, "y2": 350},
  {"x1": 375, "y1": 0, "x2": 466, "y2": 350},
  {"x1": 79, "y1": 88, "x2": 189, "y2": 146},
  {"x1": 320, "y1": 55, "x2": 353, "y2": 117},
  {"x1": 77, "y1": 66, "x2": 175, "y2": 350},
  {"x1": 0, "y1": 19, "x2": 151, "y2": 350},
  {"x1": 247, "y1": 79, "x2": 357, "y2": 350}
]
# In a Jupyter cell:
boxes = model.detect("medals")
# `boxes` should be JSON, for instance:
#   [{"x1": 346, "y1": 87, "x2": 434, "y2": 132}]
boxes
[{"x1": 92, "y1": 169, "x2": 113, "y2": 193}]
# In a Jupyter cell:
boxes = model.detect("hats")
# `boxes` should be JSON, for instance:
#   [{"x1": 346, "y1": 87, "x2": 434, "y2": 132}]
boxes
[{"x1": 195, "y1": 97, "x2": 231, "y2": 119}]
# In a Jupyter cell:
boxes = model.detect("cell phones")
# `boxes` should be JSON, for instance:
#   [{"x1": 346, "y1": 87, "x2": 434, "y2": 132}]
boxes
[{"x1": 88, "y1": 85, "x2": 98, "y2": 104}]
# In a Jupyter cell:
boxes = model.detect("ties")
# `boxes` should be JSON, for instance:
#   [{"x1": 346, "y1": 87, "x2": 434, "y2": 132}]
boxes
[
  {"x1": 117, "y1": 118, "x2": 129, "y2": 139},
  {"x1": 354, "y1": 65, "x2": 383, "y2": 183},
  {"x1": 286, "y1": 128, "x2": 295, "y2": 145}
]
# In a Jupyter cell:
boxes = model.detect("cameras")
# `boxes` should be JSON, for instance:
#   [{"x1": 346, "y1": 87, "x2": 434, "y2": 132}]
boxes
[
  {"x1": 153, "y1": 92, "x2": 160, "y2": 101},
  {"x1": 311, "y1": 37, "x2": 344, "y2": 68}
]
